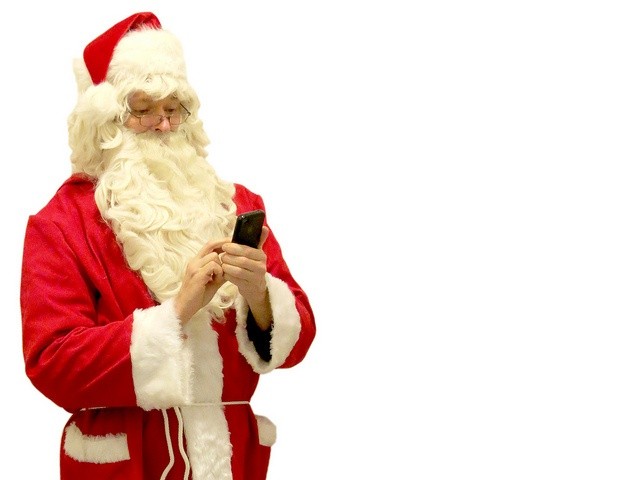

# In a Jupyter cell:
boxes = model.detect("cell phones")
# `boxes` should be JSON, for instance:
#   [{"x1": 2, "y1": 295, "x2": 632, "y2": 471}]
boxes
[{"x1": 231, "y1": 209, "x2": 265, "y2": 249}]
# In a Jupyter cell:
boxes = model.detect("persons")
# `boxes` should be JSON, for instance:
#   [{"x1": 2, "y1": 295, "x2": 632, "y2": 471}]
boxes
[{"x1": 20, "y1": 12, "x2": 318, "y2": 480}]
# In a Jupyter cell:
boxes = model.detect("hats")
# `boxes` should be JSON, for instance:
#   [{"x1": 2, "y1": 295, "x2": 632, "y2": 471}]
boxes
[{"x1": 73, "y1": 12, "x2": 186, "y2": 125}]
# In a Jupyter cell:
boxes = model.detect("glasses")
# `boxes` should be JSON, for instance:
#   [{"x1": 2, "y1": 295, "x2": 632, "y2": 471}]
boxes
[{"x1": 128, "y1": 102, "x2": 191, "y2": 127}]
